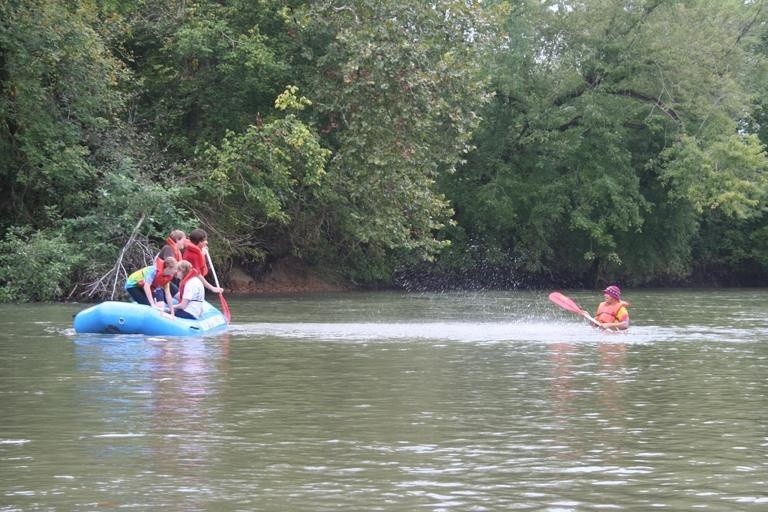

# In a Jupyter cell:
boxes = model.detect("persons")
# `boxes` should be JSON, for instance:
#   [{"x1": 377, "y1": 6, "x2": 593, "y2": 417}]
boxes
[
  {"x1": 165, "y1": 259, "x2": 205, "y2": 320},
  {"x1": 125, "y1": 256, "x2": 178, "y2": 315},
  {"x1": 181, "y1": 229, "x2": 224, "y2": 299},
  {"x1": 589, "y1": 285, "x2": 630, "y2": 332},
  {"x1": 153, "y1": 229, "x2": 186, "y2": 305}
]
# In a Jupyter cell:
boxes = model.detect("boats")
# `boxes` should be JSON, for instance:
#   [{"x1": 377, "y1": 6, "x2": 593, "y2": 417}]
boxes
[{"x1": 72, "y1": 299, "x2": 231, "y2": 336}]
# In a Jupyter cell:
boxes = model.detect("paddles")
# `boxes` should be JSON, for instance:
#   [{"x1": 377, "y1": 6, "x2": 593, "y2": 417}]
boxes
[
  {"x1": 204, "y1": 246, "x2": 231, "y2": 322},
  {"x1": 550, "y1": 292, "x2": 612, "y2": 330}
]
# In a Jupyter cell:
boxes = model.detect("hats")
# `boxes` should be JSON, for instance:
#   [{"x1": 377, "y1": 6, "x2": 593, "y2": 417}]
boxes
[{"x1": 601, "y1": 285, "x2": 619, "y2": 300}]
